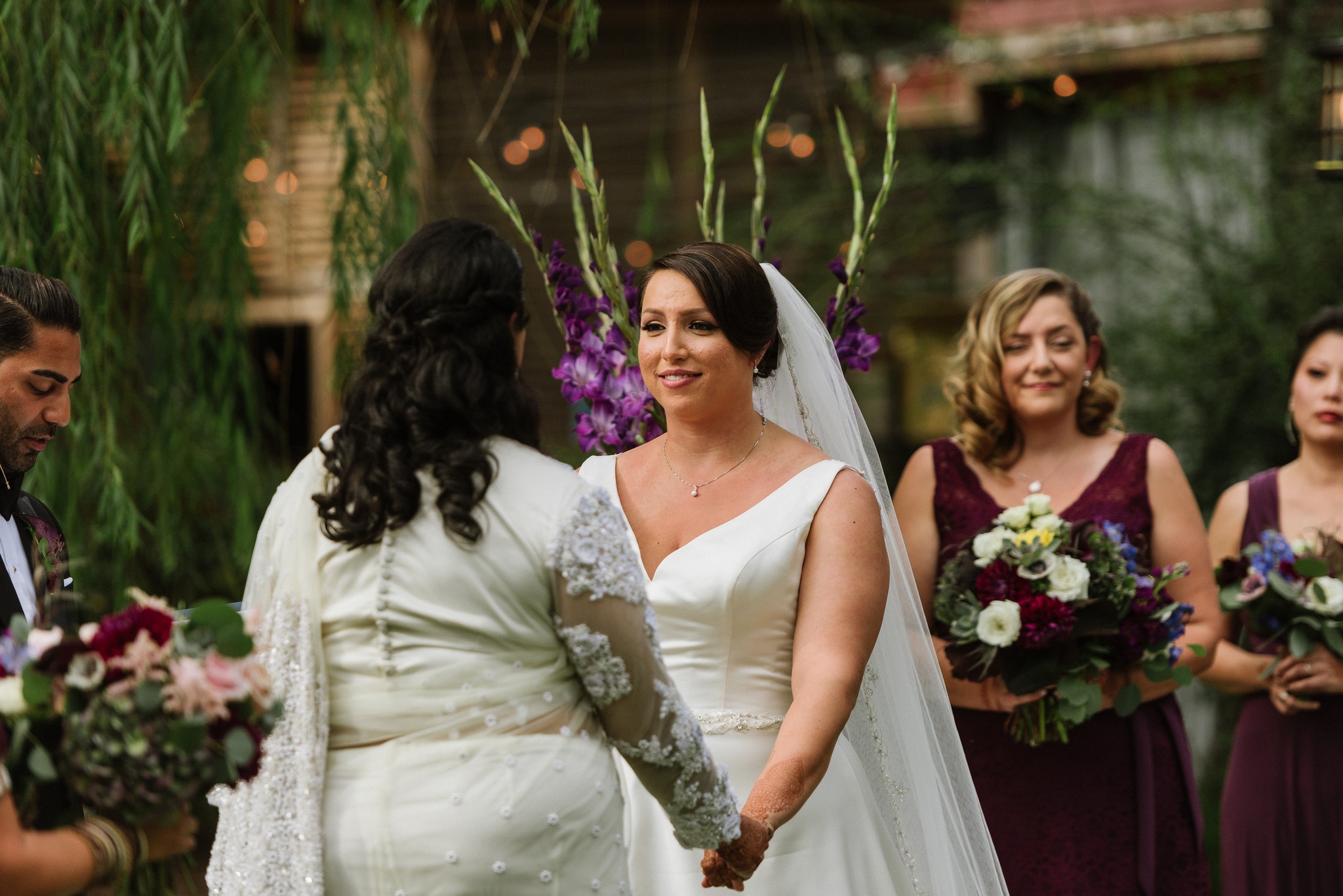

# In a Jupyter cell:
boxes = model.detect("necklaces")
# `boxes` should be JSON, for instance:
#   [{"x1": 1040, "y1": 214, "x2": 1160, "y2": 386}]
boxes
[
  {"x1": 1018, "y1": 455, "x2": 1064, "y2": 493},
  {"x1": 663, "y1": 411, "x2": 768, "y2": 496}
]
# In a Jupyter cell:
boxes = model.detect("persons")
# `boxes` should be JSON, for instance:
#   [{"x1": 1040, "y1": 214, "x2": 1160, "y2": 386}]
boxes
[
  {"x1": 581, "y1": 244, "x2": 1009, "y2": 895},
  {"x1": 1185, "y1": 302, "x2": 1343, "y2": 895},
  {"x1": 205, "y1": 219, "x2": 770, "y2": 895},
  {"x1": 895, "y1": 268, "x2": 1222, "y2": 896},
  {"x1": 0, "y1": 738, "x2": 195, "y2": 896},
  {"x1": 0, "y1": 266, "x2": 89, "y2": 747}
]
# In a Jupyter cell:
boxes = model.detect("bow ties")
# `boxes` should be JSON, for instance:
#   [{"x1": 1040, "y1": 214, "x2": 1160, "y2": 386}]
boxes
[{"x1": 0, "y1": 476, "x2": 24, "y2": 521}]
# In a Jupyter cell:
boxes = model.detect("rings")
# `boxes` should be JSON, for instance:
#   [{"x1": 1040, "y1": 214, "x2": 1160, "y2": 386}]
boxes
[
  {"x1": 1280, "y1": 693, "x2": 1288, "y2": 699},
  {"x1": 717, "y1": 875, "x2": 725, "y2": 881},
  {"x1": 1304, "y1": 663, "x2": 1312, "y2": 676}
]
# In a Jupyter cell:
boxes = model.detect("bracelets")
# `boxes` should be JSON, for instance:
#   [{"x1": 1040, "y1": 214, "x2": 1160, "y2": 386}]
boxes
[{"x1": 70, "y1": 813, "x2": 152, "y2": 883}]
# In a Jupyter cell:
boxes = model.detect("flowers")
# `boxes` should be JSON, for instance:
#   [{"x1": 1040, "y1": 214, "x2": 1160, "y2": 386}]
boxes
[
  {"x1": 931, "y1": 482, "x2": 1208, "y2": 750},
  {"x1": 0, "y1": 534, "x2": 293, "y2": 817},
  {"x1": 1211, "y1": 522, "x2": 1343, "y2": 682},
  {"x1": 527, "y1": 220, "x2": 888, "y2": 456}
]
